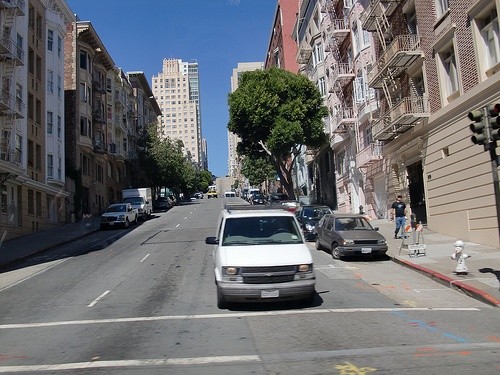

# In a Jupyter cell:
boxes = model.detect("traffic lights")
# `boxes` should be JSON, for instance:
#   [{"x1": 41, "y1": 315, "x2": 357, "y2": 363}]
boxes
[{"x1": 467, "y1": 103, "x2": 500, "y2": 152}]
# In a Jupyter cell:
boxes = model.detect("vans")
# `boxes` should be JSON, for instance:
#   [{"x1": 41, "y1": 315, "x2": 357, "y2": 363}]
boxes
[{"x1": 205, "y1": 204, "x2": 317, "y2": 309}]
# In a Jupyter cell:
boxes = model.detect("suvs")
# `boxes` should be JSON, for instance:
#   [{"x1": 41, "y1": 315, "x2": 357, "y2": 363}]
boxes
[{"x1": 240, "y1": 188, "x2": 267, "y2": 205}]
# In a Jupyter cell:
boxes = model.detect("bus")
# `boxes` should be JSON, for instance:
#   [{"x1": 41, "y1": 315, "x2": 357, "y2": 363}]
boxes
[{"x1": 206, "y1": 190, "x2": 236, "y2": 199}]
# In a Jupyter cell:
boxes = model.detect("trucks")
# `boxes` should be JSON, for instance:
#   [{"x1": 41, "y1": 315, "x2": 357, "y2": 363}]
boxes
[{"x1": 119, "y1": 188, "x2": 153, "y2": 220}]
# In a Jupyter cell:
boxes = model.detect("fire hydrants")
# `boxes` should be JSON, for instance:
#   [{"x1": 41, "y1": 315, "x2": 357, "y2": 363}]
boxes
[{"x1": 450, "y1": 240, "x2": 471, "y2": 275}]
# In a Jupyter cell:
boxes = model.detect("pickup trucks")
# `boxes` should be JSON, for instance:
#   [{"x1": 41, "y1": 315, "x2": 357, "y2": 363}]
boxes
[{"x1": 100, "y1": 203, "x2": 138, "y2": 231}]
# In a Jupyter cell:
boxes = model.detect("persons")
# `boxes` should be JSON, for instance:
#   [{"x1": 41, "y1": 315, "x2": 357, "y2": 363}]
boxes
[{"x1": 391, "y1": 196, "x2": 407, "y2": 239}]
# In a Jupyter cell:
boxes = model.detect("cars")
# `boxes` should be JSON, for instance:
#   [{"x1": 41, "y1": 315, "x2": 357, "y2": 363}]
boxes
[
  {"x1": 314, "y1": 213, "x2": 388, "y2": 260},
  {"x1": 294, "y1": 204, "x2": 341, "y2": 242},
  {"x1": 154, "y1": 195, "x2": 176, "y2": 210}
]
